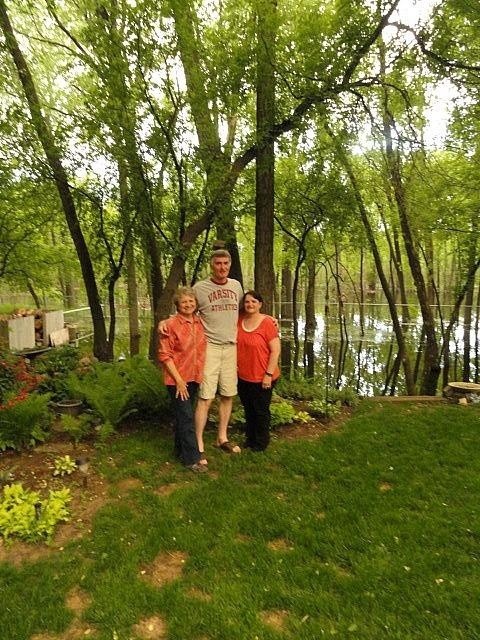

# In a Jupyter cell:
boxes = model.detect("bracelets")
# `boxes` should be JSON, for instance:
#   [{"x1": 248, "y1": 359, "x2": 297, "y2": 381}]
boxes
[{"x1": 265, "y1": 370, "x2": 273, "y2": 378}]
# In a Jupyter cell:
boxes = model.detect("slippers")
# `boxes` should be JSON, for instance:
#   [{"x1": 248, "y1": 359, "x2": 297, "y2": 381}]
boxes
[
  {"x1": 199, "y1": 450, "x2": 211, "y2": 469},
  {"x1": 212, "y1": 440, "x2": 242, "y2": 455}
]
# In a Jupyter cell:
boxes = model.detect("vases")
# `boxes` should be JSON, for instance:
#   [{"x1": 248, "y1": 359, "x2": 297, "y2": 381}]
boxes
[{"x1": 56, "y1": 398, "x2": 84, "y2": 418}]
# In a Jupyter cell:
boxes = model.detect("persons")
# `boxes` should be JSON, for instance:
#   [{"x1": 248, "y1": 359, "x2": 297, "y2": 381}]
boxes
[
  {"x1": 157, "y1": 248, "x2": 281, "y2": 467},
  {"x1": 154, "y1": 284, "x2": 209, "y2": 475},
  {"x1": 236, "y1": 288, "x2": 279, "y2": 456}
]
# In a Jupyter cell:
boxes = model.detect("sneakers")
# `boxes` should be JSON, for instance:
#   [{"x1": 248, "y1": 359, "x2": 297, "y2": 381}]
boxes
[
  {"x1": 243, "y1": 435, "x2": 271, "y2": 453},
  {"x1": 186, "y1": 464, "x2": 209, "y2": 474}
]
